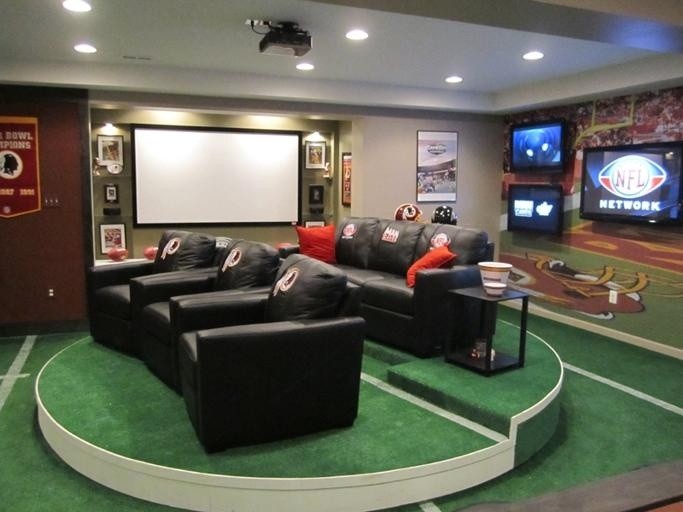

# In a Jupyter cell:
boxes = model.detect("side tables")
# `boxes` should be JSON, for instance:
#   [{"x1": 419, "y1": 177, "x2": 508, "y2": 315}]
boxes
[{"x1": 445, "y1": 285, "x2": 529, "y2": 377}]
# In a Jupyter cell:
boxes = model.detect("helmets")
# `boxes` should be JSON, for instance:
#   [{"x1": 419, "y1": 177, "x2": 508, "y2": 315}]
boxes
[
  {"x1": 432, "y1": 206, "x2": 457, "y2": 224},
  {"x1": 395, "y1": 204, "x2": 421, "y2": 221}
]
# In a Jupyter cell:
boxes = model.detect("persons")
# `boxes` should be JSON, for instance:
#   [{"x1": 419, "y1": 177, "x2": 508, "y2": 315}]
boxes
[
  {"x1": 108, "y1": 142, "x2": 120, "y2": 161},
  {"x1": 418, "y1": 160, "x2": 456, "y2": 194},
  {"x1": 105, "y1": 229, "x2": 121, "y2": 248},
  {"x1": 310, "y1": 148, "x2": 322, "y2": 164}
]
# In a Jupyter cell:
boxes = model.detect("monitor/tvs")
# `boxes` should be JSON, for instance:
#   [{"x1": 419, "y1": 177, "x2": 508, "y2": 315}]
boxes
[
  {"x1": 579, "y1": 141, "x2": 683, "y2": 226},
  {"x1": 510, "y1": 119, "x2": 566, "y2": 173},
  {"x1": 508, "y1": 183, "x2": 564, "y2": 236}
]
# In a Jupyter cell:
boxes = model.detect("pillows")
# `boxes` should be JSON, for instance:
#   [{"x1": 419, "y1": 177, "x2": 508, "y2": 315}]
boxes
[
  {"x1": 294, "y1": 224, "x2": 339, "y2": 264},
  {"x1": 402, "y1": 243, "x2": 459, "y2": 289}
]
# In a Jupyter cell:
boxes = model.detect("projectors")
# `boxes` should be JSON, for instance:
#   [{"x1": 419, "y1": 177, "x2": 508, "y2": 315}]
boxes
[{"x1": 259, "y1": 28, "x2": 311, "y2": 56}]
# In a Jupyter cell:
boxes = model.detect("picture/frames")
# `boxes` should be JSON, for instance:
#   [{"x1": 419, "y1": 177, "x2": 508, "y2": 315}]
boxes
[
  {"x1": 104, "y1": 183, "x2": 119, "y2": 204},
  {"x1": 96, "y1": 134, "x2": 124, "y2": 167},
  {"x1": 304, "y1": 140, "x2": 326, "y2": 170},
  {"x1": 343, "y1": 152, "x2": 351, "y2": 206},
  {"x1": 417, "y1": 130, "x2": 458, "y2": 202},
  {"x1": 97, "y1": 222, "x2": 130, "y2": 256}
]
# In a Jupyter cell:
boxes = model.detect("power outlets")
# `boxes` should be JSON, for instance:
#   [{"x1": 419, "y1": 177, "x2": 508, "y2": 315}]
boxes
[
  {"x1": 259, "y1": 21, "x2": 271, "y2": 26},
  {"x1": 244, "y1": 20, "x2": 258, "y2": 26}
]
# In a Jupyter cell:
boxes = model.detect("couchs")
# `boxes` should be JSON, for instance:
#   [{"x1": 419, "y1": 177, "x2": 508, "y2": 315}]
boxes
[
  {"x1": 90, "y1": 226, "x2": 369, "y2": 452},
  {"x1": 274, "y1": 216, "x2": 495, "y2": 362}
]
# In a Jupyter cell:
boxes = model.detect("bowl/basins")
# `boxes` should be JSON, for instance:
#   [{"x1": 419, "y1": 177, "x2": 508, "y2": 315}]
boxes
[{"x1": 483, "y1": 281, "x2": 507, "y2": 297}]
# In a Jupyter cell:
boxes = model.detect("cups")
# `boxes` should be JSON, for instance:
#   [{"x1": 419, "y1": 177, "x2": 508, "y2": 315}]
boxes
[
  {"x1": 476, "y1": 260, "x2": 512, "y2": 291},
  {"x1": 474, "y1": 341, "x2": 486, "y2": 358}
]
